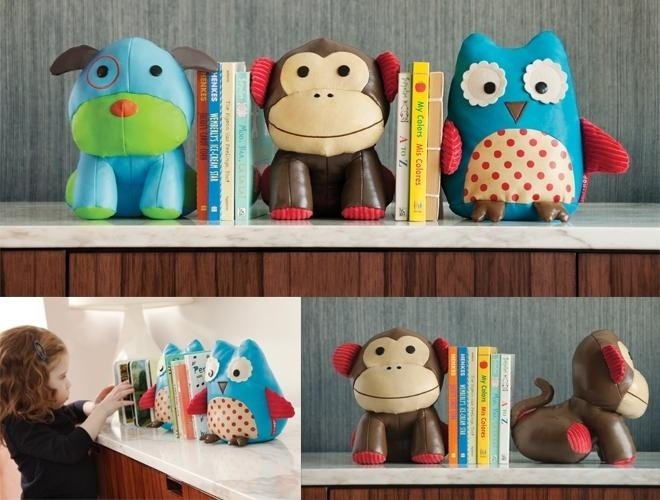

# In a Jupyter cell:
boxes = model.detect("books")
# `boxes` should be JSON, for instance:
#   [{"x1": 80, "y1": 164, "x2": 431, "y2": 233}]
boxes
[
  {"x1": 195, "y1": 60, "x2": 275, "y2": 223},
  {"x1": 446, "y1": 345, "x2": 516, "y2": 465},
  {"x1": 163, "y1": 350, "x2": 209, "y2": 441},
  {"x1": 395, "y1": 60, "x2": 446, "y2": 222},
  {"x1": 113, "y1": 357, "x2": 159, "y2": 429}
]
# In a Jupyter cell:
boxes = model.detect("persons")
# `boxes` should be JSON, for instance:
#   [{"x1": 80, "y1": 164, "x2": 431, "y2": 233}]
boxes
[{"x1": 0, "y1": 324, "x2": 135, "y2": 500}]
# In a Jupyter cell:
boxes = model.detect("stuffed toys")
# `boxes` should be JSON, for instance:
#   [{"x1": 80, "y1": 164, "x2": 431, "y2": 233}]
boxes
[
  {"x1": 331, "y1": 328, "x2": 450, "y2": 465},
  {"x1": 250, "y1": 38, "x2": 402, "y2": 221},
  {"x1": 186, "y1": 336, "x2": 295, "y2": 446},
  {"x1": 511, "y1": 328, "x2": 649, "y2": 466},
  {"x1": 138, "y1": 338, "x2": 203, "y2": 433},
  {"x1": 50, "y1": 34, "x2": 220, "y2": 220},
  {"x1": 438, "y1": 29, "x2": 629, "y2": 223}
]
